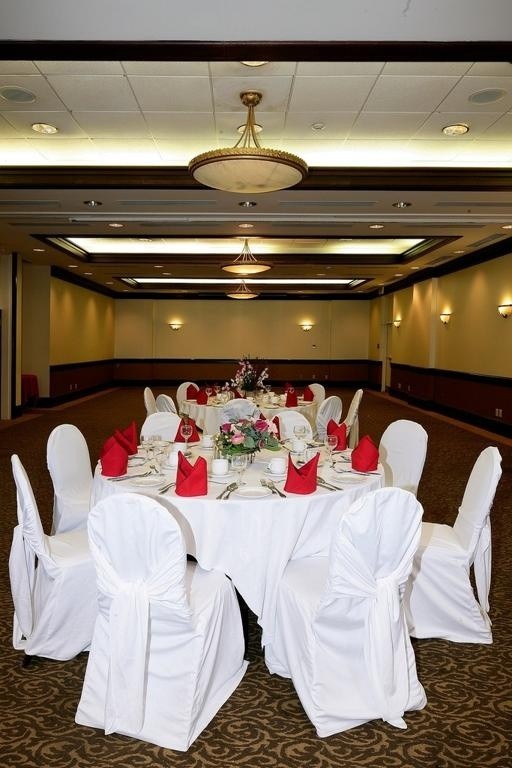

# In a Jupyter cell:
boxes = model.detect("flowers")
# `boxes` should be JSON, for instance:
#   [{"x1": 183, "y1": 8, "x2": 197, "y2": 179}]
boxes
[{"x1": 231, "y1": 351, "x2": 270, "y2": 389}]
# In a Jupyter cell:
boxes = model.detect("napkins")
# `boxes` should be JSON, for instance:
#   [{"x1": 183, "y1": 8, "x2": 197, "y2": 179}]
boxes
[
  {"x1": 176, "y1": 450, "x2": 209, "y2": 496},
  {"x1": 351, "y1": 435, "x2": 379, "y2": 473},
  {"x1": 284, "y1": 452, "x2": 319, "y2": 493},
  {"x1": 324, "y1": 419, "x2": 348, "y2": 451},
  {"x1": 115, "y1": 423, "x2": 139, "y2": 454},
  {"x1": 100, "y1": 436, "x2": 128, "y2": 478}
]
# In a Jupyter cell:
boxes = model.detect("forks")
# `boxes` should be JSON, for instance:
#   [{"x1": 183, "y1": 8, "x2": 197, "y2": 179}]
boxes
[
  {"x1": 261, "y1": 478, "x2": 285, "y2": 499},
  {"x1": 106, "y1": 440, "x2": 177, "y2": 496}
]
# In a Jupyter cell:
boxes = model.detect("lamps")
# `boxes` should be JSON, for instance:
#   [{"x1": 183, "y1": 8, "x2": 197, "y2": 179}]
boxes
[
  {"x1": 391, "y1": 321, "x2": 400, "y2": 329},
  {"x1": 218, "y1": 237, "x2": 274, "y2": 275},
  {"x1": 439, "y1": 312, "x2": 451, "y2": 326},
  {"x1": 167, "y1": 323, "x2": 183, "y2": 333},
  {"x1": 298, "y1": 323, "x2": 315, "y2": 332},
  {"x1": 184, "y1": 86, "x2": 307, "y2": 197},
  {"x1": 498, "y1": 303, "x2": 512, "y2": 319},
  {"x1": 223, "y1": 276, "x2": 261, "y2": 301}
]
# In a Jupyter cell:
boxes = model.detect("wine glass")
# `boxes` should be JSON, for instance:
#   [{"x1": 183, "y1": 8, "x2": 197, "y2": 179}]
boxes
[
  {"x1": 180, "y1": 425, "x2": 193, "y2": 453},
  {"x1": 323, "y1": 435, "x2": 339, "y2": 463}
]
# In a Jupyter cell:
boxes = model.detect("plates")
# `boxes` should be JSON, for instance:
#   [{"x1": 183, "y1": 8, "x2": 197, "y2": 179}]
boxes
[
  {"x1": 209, "y1": 470, "x2": 235, "y2": 478},
  {"x1": 296, "y1": 401, "x2": 312, "y2": 405},
  {"x1": 332, "y1": 472, "x2": 368, "y2": 484},
  {"x1": 265, "y1": 405, "x2": 279, "y2": 409},
  {"x1": 234, "y1": 487, "x2": 271, "y2": 498},
  {"x1": 261, "y1": 468, "x2": 288, "y2": 477}
]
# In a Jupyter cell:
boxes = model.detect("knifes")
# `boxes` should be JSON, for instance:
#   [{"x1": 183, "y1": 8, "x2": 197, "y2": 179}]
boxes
[
  {"x1": 216, "y1": 483, "x2": 236, "y2": 499},
  {"x1": 317, "y1": 479, "x2": 342, "y2": 492}
]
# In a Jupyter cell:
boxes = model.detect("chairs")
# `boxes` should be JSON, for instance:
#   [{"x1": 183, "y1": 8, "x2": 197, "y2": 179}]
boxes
[
  {"x1": 75, "y1": 490, "x2": 249, "y2": 753},
  {"x1": 141, "y1": 382, "x2": 363, "y2": 444},
  {"x1": 10, "y1": 454, "x2": 100, "y2": 661},
  {"x1": 264, "y1": 487, "x2": 428, "y2": 737},
  {"x1": 46, "y1": 423, "x2": 94, "y2": 535},
  {"x1": 404, "y1": 446, "x2": 503, "y2": 645},
  {"x1": 378, "y1": 419, "x2": 427, "y2": 498}
]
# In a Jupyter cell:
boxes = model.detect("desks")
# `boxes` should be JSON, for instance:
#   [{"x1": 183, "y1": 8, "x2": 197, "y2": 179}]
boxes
[{"x1": 95, "y1": 434, "x2": 386, "y2": 629}]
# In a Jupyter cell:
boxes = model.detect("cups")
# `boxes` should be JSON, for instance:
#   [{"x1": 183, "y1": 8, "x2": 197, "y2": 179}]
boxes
[
  {"x1": 294, "y1": 424, "x2": 304, "y2": 439},
  {"x1": 293, "y1": 440, "x2": 308, "y2": 452},
  {"x1": 171, "y1": 435, "x2": 228, "y2": 473},
  {"x1": 204, "y1": 384, "x2": 290, "y2": 405},
  {"x1": 268, "y1": 456, "x2": 287, "y2": 474},
  {"x1": 305, "y1": 451, "x2": 323, "y2": 464}
]
